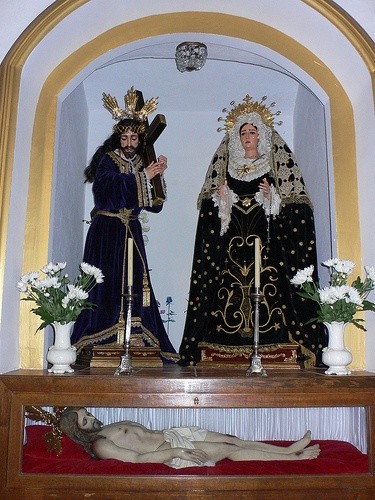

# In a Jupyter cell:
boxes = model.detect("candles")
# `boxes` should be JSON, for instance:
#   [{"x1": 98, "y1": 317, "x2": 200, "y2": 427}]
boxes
[
  {"x1": 255, "y1": 237, "x2": 260, "y2": 288},
  {"x1": 128, "y1": 236, "x2": 133, "y2": 286}
]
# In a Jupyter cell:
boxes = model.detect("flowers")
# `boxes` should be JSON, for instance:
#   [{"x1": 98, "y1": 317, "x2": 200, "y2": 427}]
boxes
[
  {"x1": 17, "y1": 261, "x2": 105, "y2": 336},
  {"x1": 155, "y1": 296, "x2": 176, "y2": 335},
  {"x1": 290, "y1": 258, "x2": 375, "y2": 332}
]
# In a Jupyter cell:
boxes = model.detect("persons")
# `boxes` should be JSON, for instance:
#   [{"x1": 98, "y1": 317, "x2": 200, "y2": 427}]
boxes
[
  {"x1": 178, "y1": 95, "x2": 329, "y2": 370},
  {"x1": 70, "y1": 86, "x2": 180, "y2": 364},
  {"x1": 58, "y1": 407, "x2": 320, "y2": 469}
]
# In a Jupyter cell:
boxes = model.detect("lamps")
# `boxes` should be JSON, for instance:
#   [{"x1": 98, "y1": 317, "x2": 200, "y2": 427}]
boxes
[{"x1": 175, "y1": 41, "x2": 208, "y2": 73}]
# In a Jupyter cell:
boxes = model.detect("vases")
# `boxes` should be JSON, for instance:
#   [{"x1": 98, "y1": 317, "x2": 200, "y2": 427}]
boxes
[
  {"x1": 321, "y1": 320, "x2": 352, "y2": 375},
  {"x1": 46, "y1": 321, "x2": 77, "y2": 374}
]
